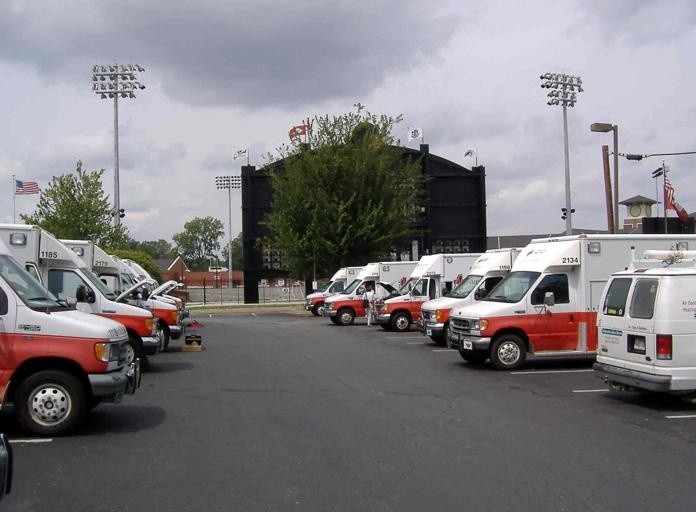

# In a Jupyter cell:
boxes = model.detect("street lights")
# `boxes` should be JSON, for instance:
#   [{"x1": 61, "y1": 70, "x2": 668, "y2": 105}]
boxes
[
  {"x1": 540, "y1": 72, "x2": 584, "y2": 238},
  {"x1": 89, "y1": 58, "x2": 145, "y2": 240},
  {"x1": 214, "y1": 175, "x2": 244, "y2": 289},
  {"x1": 591, "y1": 122, "x2": 624, "y2": 233}
]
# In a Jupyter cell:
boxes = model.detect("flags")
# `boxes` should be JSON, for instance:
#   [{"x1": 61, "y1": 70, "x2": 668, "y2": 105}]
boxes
[
  {"x1": 234, "y1": 146, "x2": 248, "y2": 160},
  {"x1": 288, "y1": 124, "x2": 305, "y2": 140},
  {"x1": 408, "y1": 128, "x2": 423, "y2": 143},
  {"x1": 664, "y1": 173, "x2": 688, "y2": 223},
  {"x1": 15, "y1": 180, "x2": 40, "y2": 195},
  {"x1": 464, "y1": 149, "x2": 475, "y2": 157}
]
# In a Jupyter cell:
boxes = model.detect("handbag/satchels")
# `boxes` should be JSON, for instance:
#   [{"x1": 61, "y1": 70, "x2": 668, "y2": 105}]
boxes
[{"x1": 363, "y1": 292, "x2": 369, "y2": 309}]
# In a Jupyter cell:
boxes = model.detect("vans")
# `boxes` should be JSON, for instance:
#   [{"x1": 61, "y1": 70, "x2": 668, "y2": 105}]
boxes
[
  {"x1": 303, "y1": 265, "x2": 364, "y2": 317},
  {"x1": 0, "y1": 221, "x2": 166, "y2": 357},
  {"x1": 322, "y1": 261, "x2": 420, "y2": 326},
  {"x1": 0, "y1": 240, "x2": 143, "y2": 437},
  {"x1": 375, "y1": 252, "x2": 485, "y2": 332},
  {"x1": 594, "y1": 249, "x2": 696, "y2": 395},
  {"x1": 422, "y1": 245, "x2": 523, "y2": 346},
  {"x1": 57, "y1": 239, "x2": 190, "y2": 341},
  {"x1": 447, "y1": 231, "x2": 696, "y2": 369}
]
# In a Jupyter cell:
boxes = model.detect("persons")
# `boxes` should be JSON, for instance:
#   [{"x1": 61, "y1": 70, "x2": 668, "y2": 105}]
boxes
[{"x1": 363, "y1": 284, "x2": 374, "y2": 326}]
